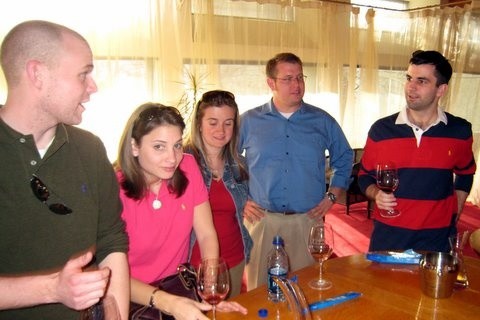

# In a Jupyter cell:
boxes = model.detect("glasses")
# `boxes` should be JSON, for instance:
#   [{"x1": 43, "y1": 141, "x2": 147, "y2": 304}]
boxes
[
  {"x1": 199, "y1": 90, "x2": 235, "y2": 103},
  {"x1": 140, "y1": 106, "x2": 180, "y2": 122},
  {"x1": 272, "y1": 75, "x2": 308, "y2": 82},
  {"x1": 30, "y1": 176, "x2": 75, "y2": 216}
]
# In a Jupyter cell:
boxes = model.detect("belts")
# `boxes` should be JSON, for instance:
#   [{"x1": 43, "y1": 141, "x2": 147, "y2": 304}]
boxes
[{"x1": 258, "y1": 207, "x2": 296, "y2": 215}]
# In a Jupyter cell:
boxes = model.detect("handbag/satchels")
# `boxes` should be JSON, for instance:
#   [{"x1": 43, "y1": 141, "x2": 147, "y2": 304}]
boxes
[{"x1": 128, "y1": 261, "x2": 202, "y2": 320}]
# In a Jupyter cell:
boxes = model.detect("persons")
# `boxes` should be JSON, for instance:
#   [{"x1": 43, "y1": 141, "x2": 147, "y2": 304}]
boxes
[
  {"x1": 112, "y1": 103, "x2": 249, "y2": 320},
  {"x1": 357, "y1": 49, "x2": 477, "y2": 253},
  {"x1": 236, "y1": 53, "x2": 354, "y2": 291},
  {"x1": 0, "y1": 19, "x2": 131, "y2": 319},
  {"x1": 183, "y1": 90, "x2": 255, "y2": 299}
]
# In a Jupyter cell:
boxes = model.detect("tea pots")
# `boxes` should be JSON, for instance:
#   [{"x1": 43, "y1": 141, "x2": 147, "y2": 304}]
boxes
[{"x1": 450, "y1": 230, "x2": 469, "y2": 299}]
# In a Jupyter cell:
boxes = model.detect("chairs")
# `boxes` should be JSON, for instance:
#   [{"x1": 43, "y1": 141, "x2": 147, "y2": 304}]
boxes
[{"x1": 322, "y1": 148, "x2": 372, "y2": 222}]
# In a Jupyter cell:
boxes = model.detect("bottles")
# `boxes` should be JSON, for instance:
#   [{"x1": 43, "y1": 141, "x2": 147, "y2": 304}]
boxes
[{"x1": 267, "y1": 236, "x2": 289, "y2": 303}]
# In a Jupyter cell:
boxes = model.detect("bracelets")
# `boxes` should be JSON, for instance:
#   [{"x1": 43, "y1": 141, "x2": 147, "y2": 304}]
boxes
[{"x1": 149, "y1": 289, "x2": 160, "y2": 308}]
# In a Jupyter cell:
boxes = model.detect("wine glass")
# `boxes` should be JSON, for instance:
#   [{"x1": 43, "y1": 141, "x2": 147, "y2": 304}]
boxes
[
  {"x1": 197, "y1": 258, "x2": 231, "y2": 320},
  {"x1": 308, "y1": 224, "x2": 334, "y2": 291},
  {"x1": 375, "y1": 161, "x2": 401, "y2": 218}
]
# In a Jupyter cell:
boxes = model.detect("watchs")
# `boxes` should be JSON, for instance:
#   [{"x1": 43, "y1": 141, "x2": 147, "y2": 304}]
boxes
[{"x1": 325, "y1": 192, "x2": 337, "y2": 202}]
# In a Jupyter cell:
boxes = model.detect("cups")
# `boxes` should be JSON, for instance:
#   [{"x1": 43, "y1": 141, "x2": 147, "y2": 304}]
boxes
[{"x1": 418, "y1": 252, "x2": 459, "y2": 298}]
